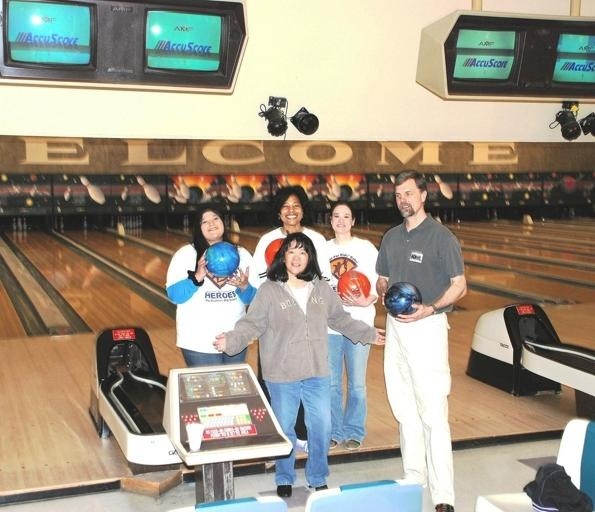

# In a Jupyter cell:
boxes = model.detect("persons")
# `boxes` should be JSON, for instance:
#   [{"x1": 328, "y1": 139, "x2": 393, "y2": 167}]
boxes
[
  {"x1": 252, "y1": 184, "x2": 333, "y2": 453},
  {"x1": 166, "y1": 203, "x2": 258, "y2": 369},
  {"x1": 327, "y1": 199, "x2": 380, "y2": 451},
  {"x1": 375, "y1": 169, "x2": 467, "y2": 512},
  {"x1": 212, "y1": 232, "x2": 386, "y2": 497}
]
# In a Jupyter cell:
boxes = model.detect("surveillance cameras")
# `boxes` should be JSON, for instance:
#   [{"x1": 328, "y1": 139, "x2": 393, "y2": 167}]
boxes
[
  {"x1": 259, "y1": 96, "x2": 289, "y2": 136},
  {"x1": 549, "y1": 101, "x2": 581, "y2": 141}
]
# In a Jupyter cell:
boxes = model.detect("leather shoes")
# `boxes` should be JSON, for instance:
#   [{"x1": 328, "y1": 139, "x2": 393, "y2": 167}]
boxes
[
  {"x1": 329, "y1": 439, "x2": 337, "y2": 449},
  {"x1": 344, "y1": 440, "x2": 360, "y2": 450},
  {"x1": 277, "y1": 485, "x2": 292, "y2": 498},
  {"x1": 436, "y1": 503, "x2": 454, "y2": 512}
]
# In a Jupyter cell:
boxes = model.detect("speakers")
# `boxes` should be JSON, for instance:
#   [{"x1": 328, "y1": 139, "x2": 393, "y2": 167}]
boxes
[
  {"x1": 581, "y1": 112, "x2": 595, "y2": 138},
  {"x1": 291, "y1": 107, "x2": 319, "y2": 135}
]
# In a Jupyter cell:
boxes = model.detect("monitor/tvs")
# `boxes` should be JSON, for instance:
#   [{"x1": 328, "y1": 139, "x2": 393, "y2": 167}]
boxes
[
  {"x1": 4, "y1": 1, "x2": 97, "y2": 71},
  {"x1": 546, "y1": 29, "x2": 595, "y2": 87},
  {"x1": 449, "y1": 25, "x2": 528, "y2": 85},
  {"x1": 144, "y1": 8, "x2": 225, "y2": 72}
]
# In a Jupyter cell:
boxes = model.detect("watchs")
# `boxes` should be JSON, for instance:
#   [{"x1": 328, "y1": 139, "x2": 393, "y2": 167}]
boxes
[{"x1": 429, "y1": 303, "x2": 438, "y2": 312}]
